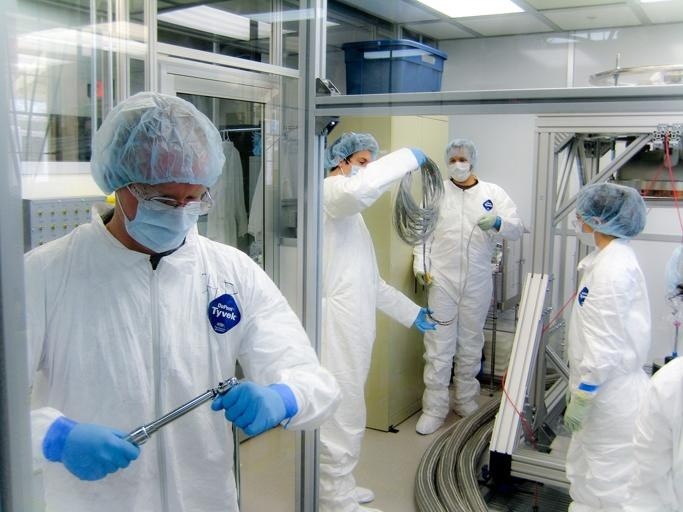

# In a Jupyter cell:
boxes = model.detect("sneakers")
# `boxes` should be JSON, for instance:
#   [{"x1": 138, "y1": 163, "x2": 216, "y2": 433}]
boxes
[
  {"x1": 452, "y1": 397, "x2": 483, "y2": 417},
  {"x1": 351, "y1": 486, "x2": 382, "y2": 512},
  {"x1": 415, "y1": 412, "x2": 446, "y2": 435}
]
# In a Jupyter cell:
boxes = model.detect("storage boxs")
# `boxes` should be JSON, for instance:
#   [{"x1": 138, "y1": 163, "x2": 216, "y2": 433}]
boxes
[{"x1": 340, "y1": 38, "x2": 448, "y2": 94}]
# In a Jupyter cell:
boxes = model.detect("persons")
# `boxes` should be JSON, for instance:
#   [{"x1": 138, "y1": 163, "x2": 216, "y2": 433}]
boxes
[
  {"x1": 559, "y1": 182, "x2": 651, "y2": 511},
  {"x1": 316, "y1": 131, "x2": 436, "y2": 512},
  {"x1": 413, "y1": 139, "x2": 524, "y2": 435},
  {"x1": 626, "y1": 243, "x2": 683, "y2": 512},
  {"x1": 23, "y1": 93, "x2": 342, "y2": 511}
]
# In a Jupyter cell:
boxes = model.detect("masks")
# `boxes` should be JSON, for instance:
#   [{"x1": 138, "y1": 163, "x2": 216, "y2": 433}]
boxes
[
  {"x1": 114, "y1": 185, "x2": 202, "y2": 255},
  {"x1": 338, "y1": 157, "x2": 363, "y2": 177},
  {"x1": 573, "y1": 216, "x2": 601, "y2": 248},
  {"x1": 449, "y1": 161, "x2": 472, "y2": 183}
]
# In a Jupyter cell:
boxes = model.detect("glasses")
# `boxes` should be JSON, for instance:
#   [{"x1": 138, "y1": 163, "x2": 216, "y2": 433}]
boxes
[{"x1": 133, "y1": 184, "x2": 214, "y2": 216}]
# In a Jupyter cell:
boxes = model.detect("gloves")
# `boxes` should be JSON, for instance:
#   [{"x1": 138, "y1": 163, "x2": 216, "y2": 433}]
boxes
[
  {"x1": 560, "y1": 390, "x2": 593, "y2": 433},
  {"x1": 414, "y1": 271, "x2": 434, "y2": 287},
  {"x1": 210, "y1": 381, "x2": 298, "y2": 437},
  {"x1": 40, "y1": 416, "x2": 142, "y2": 482},
  {"x1": 478, "y1": 214, "x2": 497, "y2": 231},
  {"x1": 410, "y1": 147, "x2": 428, "y2": 166},
  {"x1": 415, "y1": 306, "x2": 437, "y2": 334}
]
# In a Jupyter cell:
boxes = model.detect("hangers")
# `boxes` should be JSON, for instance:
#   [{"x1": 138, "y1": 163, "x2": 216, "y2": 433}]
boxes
[{"x1": 219, "y1": 128, "x2": 232, "y2": 145}]
